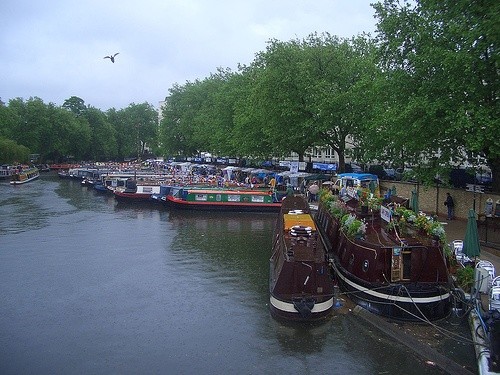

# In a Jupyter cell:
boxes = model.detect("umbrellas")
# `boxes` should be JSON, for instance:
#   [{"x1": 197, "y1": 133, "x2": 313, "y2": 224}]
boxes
[
  {"x1": 322, "y1": 181, "x2": 334, "y2": 184},
  {"x1": 409, "y1": 186, "x2": 419, "y2": 213},
  {"x1": 462, "y1": 208, "x2": 481, "y2": 266},
  {"x1": 308, "y1": 185, "x2": 320, "y2": 194}
]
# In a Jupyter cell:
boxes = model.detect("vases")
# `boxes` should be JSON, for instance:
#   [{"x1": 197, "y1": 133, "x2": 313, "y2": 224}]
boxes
[
  {"x1": 434, "y1": 235, "x2": 441, "y2": 242},
  {"x1": 353, "y1": 234, "x2": 361, "y2": 242}
]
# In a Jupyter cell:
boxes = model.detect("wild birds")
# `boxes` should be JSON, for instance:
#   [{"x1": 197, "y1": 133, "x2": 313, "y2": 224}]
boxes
[{"x1": 103, "y1": 52, "x2": 119, "y2": 63}]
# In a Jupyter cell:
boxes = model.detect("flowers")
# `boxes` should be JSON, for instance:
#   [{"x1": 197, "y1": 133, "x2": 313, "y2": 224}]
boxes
[{"x1": 320, "y1": 191, "x2": 446, "y2": 240}]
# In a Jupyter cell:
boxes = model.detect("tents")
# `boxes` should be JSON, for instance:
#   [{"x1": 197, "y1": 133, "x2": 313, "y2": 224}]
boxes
[
  {"x1": 190, "y1": 164, "x2": 199, "y2": 168},
  {"x1": 306, "y1": 174, "x2": 330, "y2": 180},
  {"x1": 220, "y1": 165, "x2": 234, "y2": 171},
  {"x1": 203, "y1": 164, "x2": 216, "y2": 169},
  {"x1": 279, "y1": 170, "x2": 310, "y2": 178},
  {"x1": 254, "y1": 169, "x2": 273, "y2": 174},
  {"x1": 338, "y1": 172, "x2": 378, "y2": 192}
]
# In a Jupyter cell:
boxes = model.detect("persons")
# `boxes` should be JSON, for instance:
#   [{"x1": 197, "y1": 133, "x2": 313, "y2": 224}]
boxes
[
  {"x1": 443, "y1": 193, "x2": 454, "y2": 220},
  {"x1": 6, "y1": 160, "x2": 49, "y2": 174},
  {"x1": 384, "y1": 188, "x2": 391, "y2": 199},
  {"x1": 59, "y1": 161, "x2": 346, "y2": 203}
]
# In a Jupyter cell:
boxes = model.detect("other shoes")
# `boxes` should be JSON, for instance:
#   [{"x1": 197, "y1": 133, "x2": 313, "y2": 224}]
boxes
[{"x1": 446, "y1": 218, "x2": 452, "y2": 220}]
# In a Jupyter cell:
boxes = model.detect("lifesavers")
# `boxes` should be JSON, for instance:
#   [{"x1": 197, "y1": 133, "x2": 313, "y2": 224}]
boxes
[
  {"x1": 450, "y1": 289, "x2": 464, "y2": 309},
  {"x1": 290, "y1": 225, "x2": 312, "y2": 236}
]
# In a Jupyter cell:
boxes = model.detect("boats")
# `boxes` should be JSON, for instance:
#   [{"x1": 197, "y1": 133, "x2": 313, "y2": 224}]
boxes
[
  {"x1": 9, "y1": 168, "x2": 40, "y2": 184},
  {"x1": 314, "y1": 186, "x2": 455, "y2": 324},
  {"x1": 268, "y1": 195, "x2": 334, "y2": 322},
  {"x1": 57, "y1": 165, "x2": 285, "y2": 207}
]
